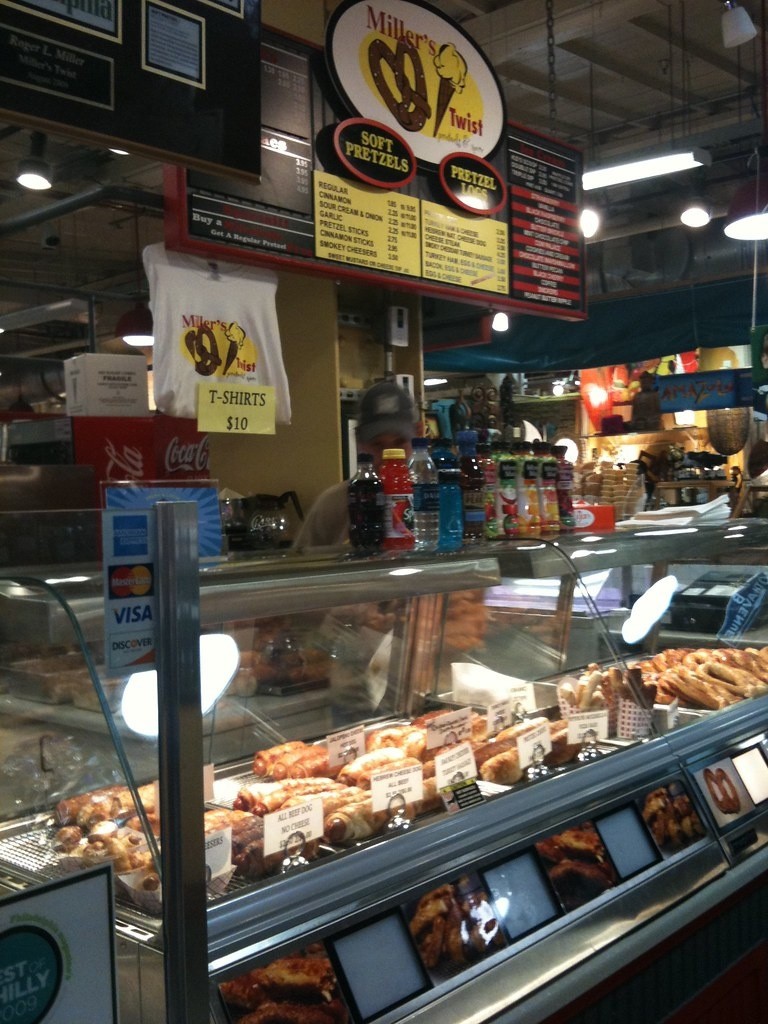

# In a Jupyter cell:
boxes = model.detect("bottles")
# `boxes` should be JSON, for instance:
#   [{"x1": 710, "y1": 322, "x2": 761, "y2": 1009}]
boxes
[
  {"x1": 476, "y1": 443, "x2": 503, "y2": 540},
  {"x1": 377, "y1": 448, "x2": 416, "y2": 551},
  {"x1": 454, "y1": 429, "x2": 486, "y2": 546},
  {"x1": 348, "y1": 453, "x2": 386, "y2": 556},
  {"x1": 408, "y1": 437, "x2": 440, "y2": 553},
  {"x1": 495, "y1": 440, "x2": 577, "y2": 538},
  {"x1": 433, "y1": 438, "x2": 463, "y2": 551}
]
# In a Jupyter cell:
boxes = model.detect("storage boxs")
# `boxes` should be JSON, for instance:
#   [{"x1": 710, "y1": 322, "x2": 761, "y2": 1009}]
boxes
[{"x1": 9, "y1": 355, "x2": 217, "y2": 561}]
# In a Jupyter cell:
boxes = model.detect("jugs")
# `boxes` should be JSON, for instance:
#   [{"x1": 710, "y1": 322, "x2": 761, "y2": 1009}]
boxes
[{"x1": 239, "y1": 489, "x2": 304, "y2": 551}]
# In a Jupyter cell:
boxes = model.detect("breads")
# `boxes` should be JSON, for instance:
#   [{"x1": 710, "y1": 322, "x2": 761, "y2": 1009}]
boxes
[
  {"x1": 52, "y1": 706, "x2": 587, "y2": 891},
  {"x1": 642, "y1": 787, "x2": 706, "y2": 850},
  {"x1": 535, "y1": 830, "x2": 617, "y2": 914},
  {"x1": 703, "y1": 767, "x2": 740, "y2": 815},
  {"x1": 216, "y1": 953, "x2": 353, "y2": 1024},
  {"x1": 200, "y1": 578, "x2": 489, "y2": 700},
  {"x1": 557, "y1": 645, "x2": 768, "y2": 716},
  {"x1": 406, "y1": 881, "x2": 501, "y2": 970}
]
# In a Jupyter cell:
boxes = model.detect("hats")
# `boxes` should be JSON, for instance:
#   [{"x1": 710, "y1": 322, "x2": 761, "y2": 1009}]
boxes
[{"x1": 358, "y1": 381, "x2": 413, "y2": 441}]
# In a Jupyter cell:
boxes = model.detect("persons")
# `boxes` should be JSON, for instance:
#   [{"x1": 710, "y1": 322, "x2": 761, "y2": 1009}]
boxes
[{"x1": 292, "y1": 382, "x2": 423, "y2": 720}]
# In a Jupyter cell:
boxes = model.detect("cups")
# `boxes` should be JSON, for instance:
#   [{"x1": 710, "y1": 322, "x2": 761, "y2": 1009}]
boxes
[{"x1": 218, "y1": 498, "x2": 249, "y2": 552}]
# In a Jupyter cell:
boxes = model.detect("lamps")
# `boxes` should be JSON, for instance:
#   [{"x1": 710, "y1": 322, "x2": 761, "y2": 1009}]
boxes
[
  {"x1": 722, "y1": 173, "x2": 768, "y2": 242},
  {"x1": 492, "y1": 311, "x2": 510, "y2": 333},
  {"x1": 583, "y1": 146, "x2": 711, "y2": 193},
  {"x1": 717, "y1": 0, "x2": 759, "y2": 49},
  {"x1": 16, "y1": 129, "x2": 58, "y2": 193},
  {"x1": 677, "y1": 186, "x2": 715, "y2": 230}
]
